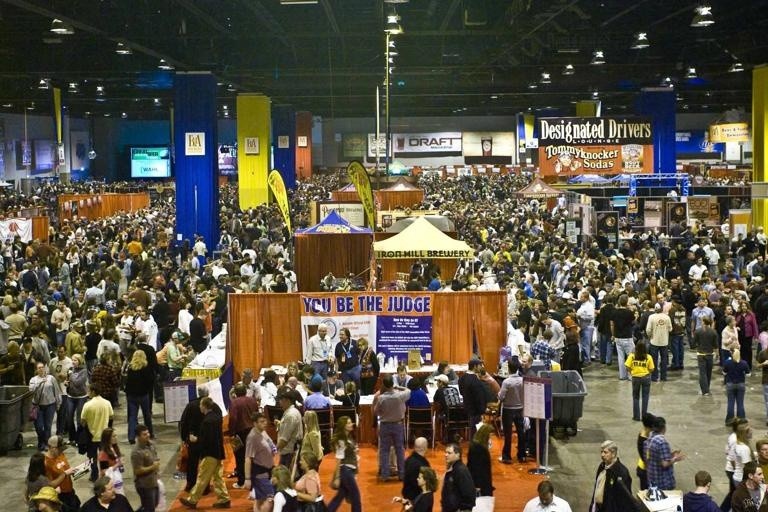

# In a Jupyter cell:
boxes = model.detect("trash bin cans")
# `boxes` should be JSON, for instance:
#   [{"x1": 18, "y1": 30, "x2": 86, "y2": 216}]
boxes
[
  {"x1": 537, "y1": 370, "x2": 587, "y2": 439},
  {"x1": 1, "y1": 384, "x2": 33, "y2": 455}
]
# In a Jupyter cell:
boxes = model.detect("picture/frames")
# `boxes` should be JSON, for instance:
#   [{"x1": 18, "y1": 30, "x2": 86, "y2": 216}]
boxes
[{"x1": 69, "y1": 129, "x2": 90, "y2": 172}]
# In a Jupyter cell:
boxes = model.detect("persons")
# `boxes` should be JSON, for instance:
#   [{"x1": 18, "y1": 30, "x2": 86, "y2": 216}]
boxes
[
  {"x1": 522, "y1": 352, "x2": 538, "y2": 458},
  {"x1": 498, "y1": 357, "x2": 526, "y2": 463},
  {"x1": 635, "y1": 414, "x2": 661, "y2": 490},
  {"x1": 477, "y1": 365, "x2": 501, "y2": 403},
  {"x1": 457, "y1": 358, "x2": 487, "y2": 442},
  {"x1": 594, "y1": 219, "x2": 768, "y2": 425},
  {"x1": 391, "y1": 466, "x2": 438, "y2": 512},
  {"x1": 718, "y1": 418, "x2": 745, "y2": 511},
  {"x1": 588, "y1": 440, "x2": 632, "y2": 512},
  {"x1": 643, "y1": 418, "x2": 684, "y2": 492},
  {"x1": 406, "y1": 379, "x2": 431, "y2": 409},
  {"x1": 733, "y1": 424, "x2": 753, "y2": 485},
  {"x1": 469, "y1": 408, "x2": 494, "y2": 494},
  {"x1": 754, "y1": 440, "x2": 768, "y2": 481},
  {"x1": 684, "y1": 470, "x2": 721, "y2": 512},
  {"x1": 441, "y1": 444, "x2": 475, "y2": 512},
  {"x1": 521, "y1": 482, "x2": 573, "y2": 512},
  {"x1": 435, "y1": 375, "x2": 461, "y2": 422},
  {"x1": 705, "y1": 175, "x2": 750, "y2": 187},
  {"x1": 392, "y1": 365, "x2": 412, "y2": 390},
  {"x1": 433, "y1": 360, "x2": 459, "y2": 385},
  {"x1": 402, "y1": 437, "x2": 430, "y2": 500},
  {"x1": 408, "y1": 173, "x2": 595, "y2": 369},
  {"x1": 731, "y1": 461, "x2": 768, "y2": 511},
  {"x1": 1, "y1": 172, "x2": 377, "y2": 511},
  {"x1": 371, "y1": 375, "x2": 412, "y2": 481}
]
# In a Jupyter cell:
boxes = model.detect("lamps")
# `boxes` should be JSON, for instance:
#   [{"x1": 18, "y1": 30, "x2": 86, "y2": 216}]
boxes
[
  {"x1": 382, "y1": 0, "x2": 403, "y2": 98},
  {"x1": 115, "y1": 40, "x2": 129, "y2": 53},
  {"x1": 50, "y1": 18, "x2": 67, "y2": 32},
  {"x1": 38, "y1": 77, "x2": 106, "y2": 96},
  {"x1": 526, "y1": 0, "x2": 745, "y2": 89},
  {"x1": 158, "y1": 58, "x2": 170, "y2": 69}
]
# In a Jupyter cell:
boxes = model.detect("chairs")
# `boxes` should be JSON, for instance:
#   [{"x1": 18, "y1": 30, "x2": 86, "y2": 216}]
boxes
[
  {"x1": 440, "y1": 402, "x2": 471, "y2": 445},
  {"x1": 480, "y1": 395, "x2": 501, "y2": 439},
  {"x1": 266, "y1": 404, "x2": 285, "y2": 441},
  {"x1": 305, "y1": 404, "x2": 333, "y2": 441},
  {"x1": 377, "y1": 412, "x2": 407, "y2": 447},
  {"x1": 406, "y1": 405, "x2": 437, "y2": 449},
  {"x1": 332, "y1": 405, "x2": 362, "y2": 441}
]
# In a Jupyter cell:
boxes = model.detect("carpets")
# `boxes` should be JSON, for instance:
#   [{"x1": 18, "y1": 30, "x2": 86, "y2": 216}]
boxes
[{"x1": 168, "y1": 412, "x2": 549, "y2": 512}]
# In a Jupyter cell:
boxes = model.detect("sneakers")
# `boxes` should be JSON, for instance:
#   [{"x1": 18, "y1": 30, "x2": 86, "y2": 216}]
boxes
[
  {"x1": 212, "y1": 500, "x2": 230, "y2": 508},
  {"x1": 699, "y1": 390, "x2": 709, "y2": 395},
  {"x1": 518, "y1": 456, "x2": 526, "y2": 462},
  {"x1": 232, "y1": 483, "x2": 245, "y2": 489},
  {"x1": 499, "y1": 457, "x2": 512, "y2": 464},
  {"x1": 226, "y1": 473, "x2": 236, "y2": 478},
  {"x1": 179, "y1": 497, "x2": 196, "y2": 509}
]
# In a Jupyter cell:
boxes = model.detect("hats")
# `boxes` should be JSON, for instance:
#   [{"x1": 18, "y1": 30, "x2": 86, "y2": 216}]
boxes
[
  {"x1": 434, "y1": 374, "x2": 448, "y2": 384},
  {"x1": 242, "y1": 368, "x2": 254, "y2": 378},
  {"x1": 198, "y1": 385, "x2": 208, "y2": 396},
  {"x1": 672, "y1": 294, "x2": 683, "y2": 303},
  {"x1": 21, "y1": 337, "x2": 32, "y2": 342},
  {"x1": 655, "y1": 302, "x2": 663, "y2": 310},
  {"x1": 543, "y1": 329, "x2": 553, "y2": 339},
  {"x1": 171, "y1": 331, "x2": 185, "y2": 341},
  {"x1": 697, "y1": 297, "x2": 705, "y2": 301},
  {"x1": 73, "y1": 321, "x2": 83, "y2": 328},
  {"x1": 598, "y1": 290, "x2": 606, "y2": 300},
  {"x1": 327, "y1": 371, "x2": 336, "y2": 376},
  {"x1": 469, "y1": 359, "x2": 483, "y2": 365},
  {"x1": 275, "y1": 385, "x2": 293, "y2": 399},
  {"x1": 288, "y1": 377, "x2": 300, "y2": 385},
  {"x1": 29, "y1": 486, "x2": 63, "y2": 506}
]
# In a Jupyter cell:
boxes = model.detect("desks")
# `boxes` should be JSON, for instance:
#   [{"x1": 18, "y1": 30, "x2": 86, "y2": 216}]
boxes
[
  {"x1": 260, "y1": 360, "x2": 470, "y2": 394},
  {"x1": 182, "y1": 323, "x2": 227, "y2": 385},
  {"x1": 327, "y1": 394, "x2": 435, "y2": 443}
]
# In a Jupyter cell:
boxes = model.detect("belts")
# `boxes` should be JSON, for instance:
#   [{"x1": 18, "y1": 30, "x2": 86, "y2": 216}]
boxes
[{"x1": 382, "y1": 422, "x2": 397, "y2": 424}]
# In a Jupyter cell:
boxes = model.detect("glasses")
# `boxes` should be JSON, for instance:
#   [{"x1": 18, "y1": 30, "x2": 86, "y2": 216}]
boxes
[{"x1": 58, "y1": 442, "x2": 66, "y2": 447}]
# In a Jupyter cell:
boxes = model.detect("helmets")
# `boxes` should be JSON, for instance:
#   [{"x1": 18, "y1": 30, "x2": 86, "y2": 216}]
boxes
[{"x1": 47, "y1": 435, "x2": 67, "y2": 451}]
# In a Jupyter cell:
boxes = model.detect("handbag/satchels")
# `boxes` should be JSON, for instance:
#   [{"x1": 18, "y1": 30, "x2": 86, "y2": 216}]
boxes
[
  {"x1": 301, "y1": 495, "x2": 327, "y2": 512},
  {"x1": 28, "y1": 406, "x2": 37, "y2": 422},
  {"x1": 176, "y1": 441, "x2": 191, "y2": 472}
]
